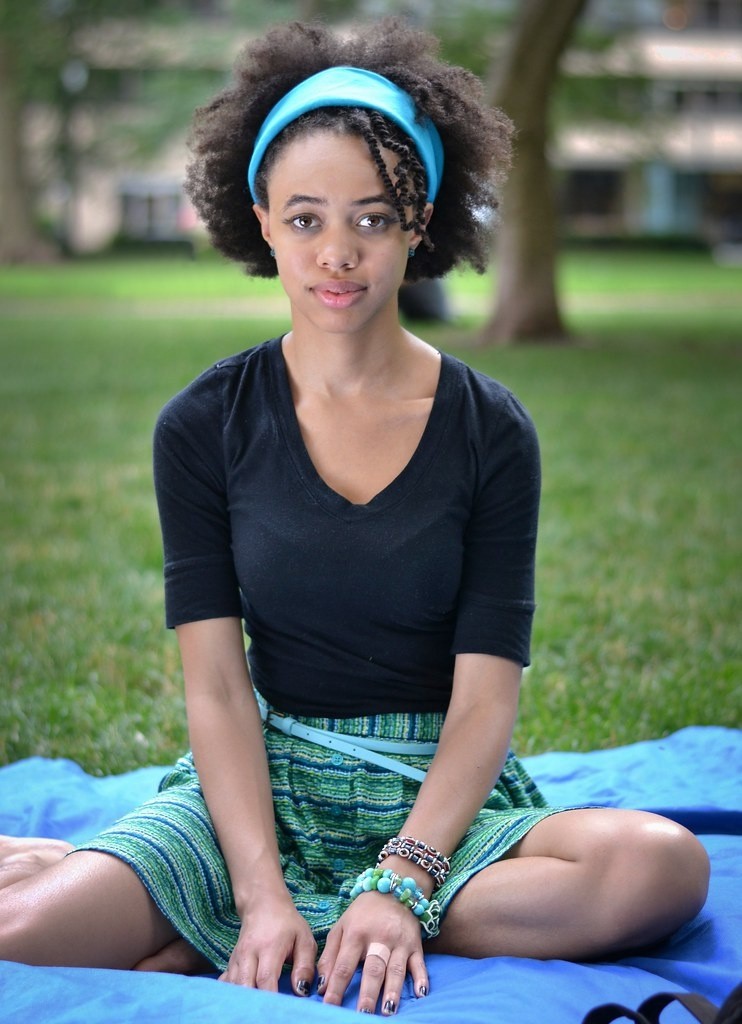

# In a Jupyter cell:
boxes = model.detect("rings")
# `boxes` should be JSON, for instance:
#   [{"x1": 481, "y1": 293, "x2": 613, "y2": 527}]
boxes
[{"x1": 367, "y1": 943, "x2": 391, "y2": 965}]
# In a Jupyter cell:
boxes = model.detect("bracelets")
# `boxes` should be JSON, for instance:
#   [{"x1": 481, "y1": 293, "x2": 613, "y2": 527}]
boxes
[
  {"x1": 350, "y1": 868, "x2": 440, "y2": 938},
  {"x1": 377, "y1": 837, "x2": 450, "y2": 888}
]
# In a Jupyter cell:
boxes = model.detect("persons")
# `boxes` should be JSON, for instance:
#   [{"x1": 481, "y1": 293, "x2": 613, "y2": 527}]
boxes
[{"x1": 0, "y1": 20, "x2": 711, "y2": 1015}]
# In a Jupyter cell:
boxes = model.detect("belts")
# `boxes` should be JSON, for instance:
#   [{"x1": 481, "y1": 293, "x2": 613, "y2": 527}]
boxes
[{"x1": 258, "y1": 705, "x2": 437, "y2": 784}]
